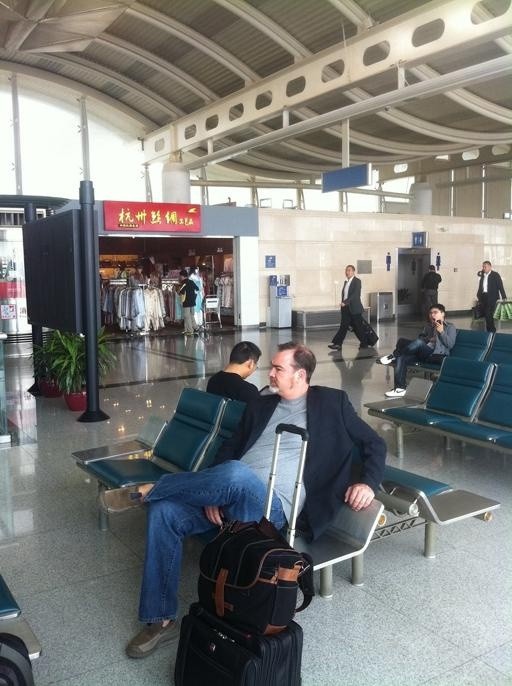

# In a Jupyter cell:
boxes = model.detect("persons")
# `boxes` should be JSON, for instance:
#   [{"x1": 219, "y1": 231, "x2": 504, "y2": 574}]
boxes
[
  {"x1": 173, "y1": 269, "x2": 200, "y2": 335},
  {"x1": 420, "y1": 264, "x2": 443, "y2": 323},
  {"x1": 205, "y1": 341, "x2": 262, "y2": 401},
  {"x1": 99, "y1": 341, "x2": 389, "y2": 659},
  {"x1": 378, "y1": 303, "x2": 457, "y2": 397},
  {"x1": 327, "y1": 264, "x2": 369, "y2": 350},
  {"x1": 476, "y1": 260, "x2": 507, "y2": 333}
]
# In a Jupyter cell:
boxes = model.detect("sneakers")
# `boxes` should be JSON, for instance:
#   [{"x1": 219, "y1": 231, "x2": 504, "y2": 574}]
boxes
[
  {"x1": 99, "y1": 484, "x2": 144, "y2": 514},
  {"x1": 127, "y1": 620, "x2": 181, "y2": 659},
  {"x1": 182, "y1": 331, "x2": 193, "y2": 335},
  {"x1": 384, "y1": 387, "x2": 406, "y2": 397},
  {"x1": 194, "y1": 328, "x2": 199, "y2": 333},
  {"x1": 379, "y1": 354, "x2": 396, "y2": 365}
]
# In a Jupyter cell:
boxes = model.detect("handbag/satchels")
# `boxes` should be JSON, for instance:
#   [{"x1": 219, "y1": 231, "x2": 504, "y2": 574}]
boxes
[
  {"x1": 198, "y1": 518, "x2": 315, "y2": 633},
  {"x1": 472, "y1": 301, "x2": 484, "y2": 320}
]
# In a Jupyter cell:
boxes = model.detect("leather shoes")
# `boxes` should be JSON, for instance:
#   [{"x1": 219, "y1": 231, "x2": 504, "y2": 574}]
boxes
[
  {"x1": 328, "y1": 344, "x2": 341, "y2": 351},
  {"x1": 360, "y1": 342, "x2": 368, "y2": 346}
]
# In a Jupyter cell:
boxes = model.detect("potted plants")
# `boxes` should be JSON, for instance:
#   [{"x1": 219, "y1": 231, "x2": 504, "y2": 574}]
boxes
[
  {"x1": 31, "y1": 332, "x2": 69, "y2": 398},
  {"x1": 55, "y1": 330, "x2": 118, "y2": 410}
]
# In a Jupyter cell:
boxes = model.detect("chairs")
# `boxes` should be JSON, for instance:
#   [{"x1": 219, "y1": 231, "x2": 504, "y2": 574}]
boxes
[
  {"x1": 88, "y1": 386, "x2": 224, "y2": 532},
  {"x1": 385, "y1": 356, "x2": 494, "y2": 460},
  {"x1": 0, "y1": 575, "x2": 22, "y2": 619},
  {"x1": 288, "y1": 496, "x2": 385, "y2": 600},
  {"x1": 124, "y1": 400, "x2": 248, "y2": 494},
  {"x1": 363, "y1": 378, "x2": 434, "y2": 412},
  {"x1": 437, "y1": 364, "x2": 511, "y2": 443},
  {"x1": 379, "y1": 464, "x2": 452, "y2": 517},
  {"x1": 72, "y1": 416, "x2": 167, "y2": 466},
  {"x1": 484, "y1": 332, "x2": 512, "y2": 363},
  {"x1": 0, "y1": 616, "x2": 42, "y2": 661},
  {"x1": 420, "y1": 329, "x2": 493, "y2": 380},
  {"x1": 390, "y1": 317, "x2": 459, "y2": 389},
  {"x1": 384, "y1": 480, "x2": 500, "y2": 560}
]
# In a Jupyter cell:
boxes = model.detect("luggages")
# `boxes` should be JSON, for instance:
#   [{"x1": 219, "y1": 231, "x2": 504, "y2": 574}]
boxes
[
  {"x1": 359, "y1": 316, "x2": 379, "y2": 346},
  {"x1": 174, "y1": 424, "x2": 308, "y2": 686}
]
